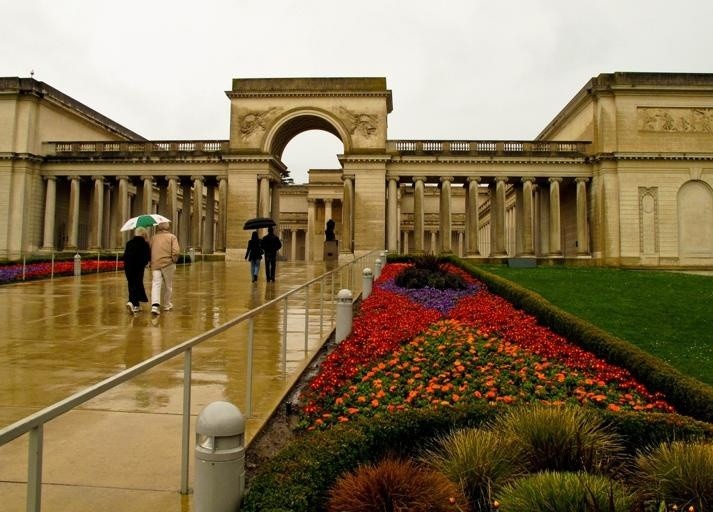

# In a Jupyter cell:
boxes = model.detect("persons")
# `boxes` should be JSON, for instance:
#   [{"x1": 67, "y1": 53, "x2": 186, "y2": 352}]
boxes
[
  {"x1": 261, "y1": 228, "x2": 281, "y2": 282},
  {"x1": 123, "y1": 226, "x2": 151, "y2": 314},
  {"x1": 244, "y1": 232, "x2": 262, "y2": 282},
  {"x1": 149, "y1": 222, "x2": 179, "y2": 315}
]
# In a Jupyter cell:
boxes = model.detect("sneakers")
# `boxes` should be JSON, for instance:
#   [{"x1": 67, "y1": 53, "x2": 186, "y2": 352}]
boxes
[
  {"x1": 250, "y1": 276, "x2": 276, "y2": 283},
  {"x1": 163, "y1": 303, "x2": 175, "y2": 312},
  {"x1": 125, "y1": 302, "x2": 135, "y2": 315},
  {"x1": 150, "y1": 306, "x2": 161, "y2": 315},
  {"x1": 134, "y1": 305, "x2": 144, "y2": 312}
]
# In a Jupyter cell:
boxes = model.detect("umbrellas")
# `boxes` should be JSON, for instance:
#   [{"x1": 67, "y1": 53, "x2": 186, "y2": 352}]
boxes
[
  {"x1": 242, "y1": 217, "x2": 276, "y2": 232},
  {"x1": 120, "y1": 214, "x2": 171, "y2": 232}
]
